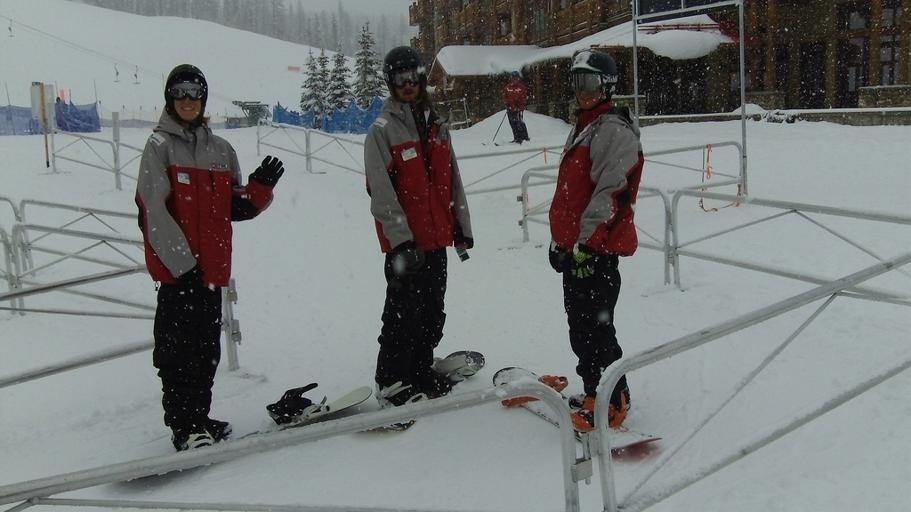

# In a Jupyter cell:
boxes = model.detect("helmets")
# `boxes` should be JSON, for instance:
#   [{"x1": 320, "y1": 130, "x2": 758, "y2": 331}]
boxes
[
  {"x1": 165, "y1": 64, "x2": 209, "y2": 120},
  {"x1": 571, "y1": 50, "x2": 618, "y2": 104},
  {"x1": 383, "y1": 45, "x2": 426, "y2": 98}
]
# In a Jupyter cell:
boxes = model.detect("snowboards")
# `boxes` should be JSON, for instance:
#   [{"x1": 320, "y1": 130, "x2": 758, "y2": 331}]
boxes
[
  {"x1": 107, "y1": 381, "x2": 372, "y2": 483},
  {"x1": 363, "y1": 349, "x2": 485, "y2": 436},
  {"x1": 491, "y1": 367, "x2": 663, "y2": 452}
]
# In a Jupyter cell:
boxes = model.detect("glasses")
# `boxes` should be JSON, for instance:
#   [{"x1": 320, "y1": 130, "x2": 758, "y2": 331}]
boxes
[
  {"x1": 573, "y1": 73, "x2": 607, "y2": 89},
  {"x1": 391, "y1": 70, "x2": 421, "y2": 88},
  {"x1": 164, "y1": 84, "x2": 208, "y2": 101}
]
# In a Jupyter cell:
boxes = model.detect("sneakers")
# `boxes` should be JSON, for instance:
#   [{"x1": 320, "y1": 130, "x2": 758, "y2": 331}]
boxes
[
  {"x1": 373, "y1": 372, "x2": 439, "y2": 408},
  {"x1": 169, "y1": 414, "x2": 232, "y2": 451},
  {"x1": 566, "y1": 386, "x2": 634, "y2": 435}
]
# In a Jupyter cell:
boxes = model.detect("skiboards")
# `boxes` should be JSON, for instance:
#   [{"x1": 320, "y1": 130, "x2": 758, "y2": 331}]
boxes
[{"x1": 480, "y1": 139, "x2": 531, "y2": 147}]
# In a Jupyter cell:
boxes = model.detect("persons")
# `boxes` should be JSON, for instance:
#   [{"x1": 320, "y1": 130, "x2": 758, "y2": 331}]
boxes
[
  {"x1": 502, "y1": 71, "x2": 528, "y2": 144},
  {"x1": 363, "y1": 47, "x2": 474, "y2": 406},
  {"x1": 549, "y1": 50, "x2": 645, "y2": 433},
  {"x1": 135, "y1": 64, "x2": 284, "y2": 452}
]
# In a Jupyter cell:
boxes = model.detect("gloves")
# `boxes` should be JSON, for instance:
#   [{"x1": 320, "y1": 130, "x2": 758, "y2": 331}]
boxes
[
  {"x1": 546, "y1": 241, "x2": 572, "y2": 274},
  {"x1": 393, "y1": 245, "x2": 422, "y2": 277},
  {"x1": 249, "y1": 156, "x2": 285, "y2": 190},
  {"x1": 178, "y1": 265, "x2": 207, "y2": 289},
  {"x1": 568, "y1": 244, "x2": 597, "y2": 281}
]
